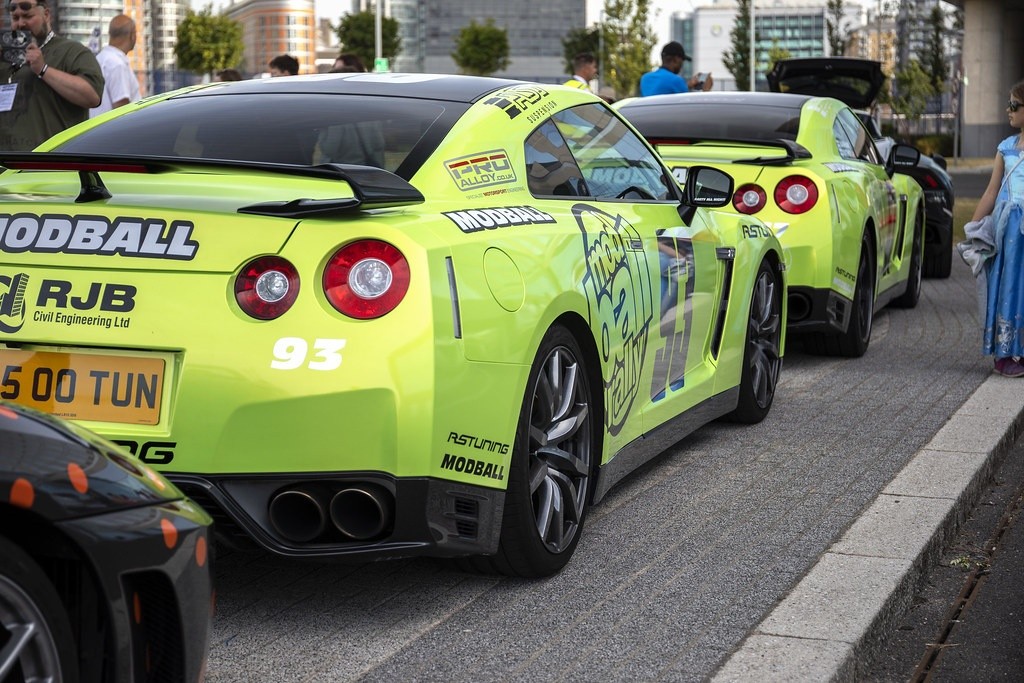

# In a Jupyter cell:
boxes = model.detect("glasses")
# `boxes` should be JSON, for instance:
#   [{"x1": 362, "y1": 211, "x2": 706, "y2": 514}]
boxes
[
  {"x1": 5, "y1": 2, "x2": 46, "y2": 12},
  {"x1": 1009, "y1": 101, "x2": 1024, "y2": 111}
]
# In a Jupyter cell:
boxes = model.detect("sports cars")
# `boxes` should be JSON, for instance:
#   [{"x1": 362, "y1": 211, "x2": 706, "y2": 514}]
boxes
[
  {"x1": 609, "y1": 90, "x2": 925, "y2": 357},
  {"x1": 0, "y1": 400, "x2": 214, "y2": 683},
  {"x1": 766, "y1": 55, "x2": 956, "y2": 281},
  {"x1": 0, "y1": 74, "x2": 787, "y2": 577}
]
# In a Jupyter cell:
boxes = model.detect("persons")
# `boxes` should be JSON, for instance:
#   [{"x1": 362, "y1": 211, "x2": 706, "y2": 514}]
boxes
[
  {"x1": 269, "y1": 55, "x2": 317, "y2": 165},
  {"x1": 197, "y1": 69, "x2": 241, "y2": 160},
  {"x1": 89, "y1": 15, "x2": 142, "y2": 119},
  {"x1": 641, "y1": 41, "x2": 713, "y2": 98},
  {"x1": 319, "y1": 53, "x2": 385, "y2": 170},
  {"x1": 969, "y1": 80, "x2": 1024, "y2": 376},
  {"x1": 0, "y1": 0, "x2": 106, "y2": 151},
  {"x1": 564, "y1": 52, "x2": 597, "y2": 93}
]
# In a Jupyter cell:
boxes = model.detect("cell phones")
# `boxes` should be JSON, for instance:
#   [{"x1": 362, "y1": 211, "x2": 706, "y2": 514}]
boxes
[
  {"x1": 0, "y1": 29, "x2": 32, "y2": 65},
  {"x1": 698, "y1": 74, "x2": 709, "y2": 81}
]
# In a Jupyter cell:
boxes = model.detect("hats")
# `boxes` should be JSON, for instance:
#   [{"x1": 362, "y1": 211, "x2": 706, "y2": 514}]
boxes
[{"x1": 662, "y1": 42, "x2": 692, "y2": 61}]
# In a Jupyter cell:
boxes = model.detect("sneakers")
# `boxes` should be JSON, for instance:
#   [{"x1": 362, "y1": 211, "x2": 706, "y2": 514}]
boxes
[{"x1": 994, "y1": 357, "x2": 1024, "y2": 377}]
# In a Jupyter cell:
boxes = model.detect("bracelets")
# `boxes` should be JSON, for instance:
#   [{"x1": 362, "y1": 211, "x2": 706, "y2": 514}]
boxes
[{"x1": 38, "y1": 64, "x2": 48, "y2": 78}]
